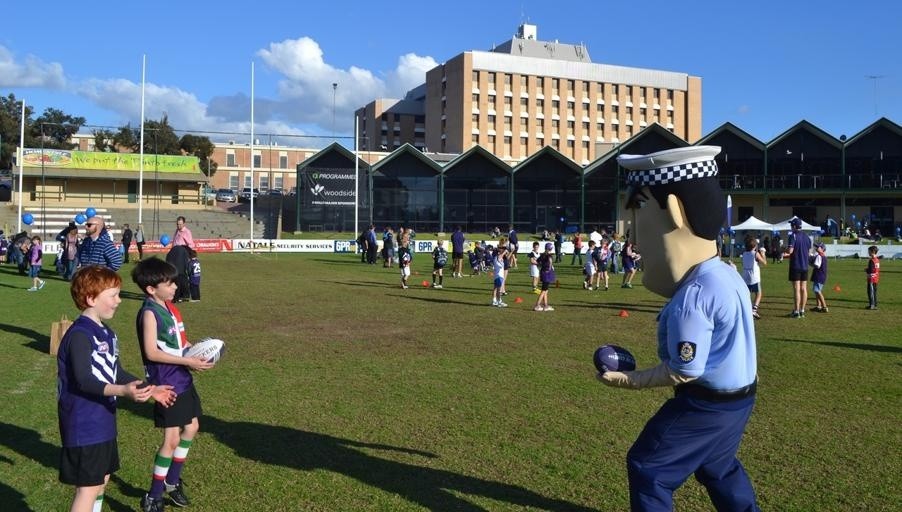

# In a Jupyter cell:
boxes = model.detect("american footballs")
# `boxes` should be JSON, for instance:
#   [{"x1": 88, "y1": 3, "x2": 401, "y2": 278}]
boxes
[
  {"x1": 593, "y1": 345, "x2": 635, "y2": 375},
  {"x1": 182, "y1": 336, "x2": 226, "y2": 370}
]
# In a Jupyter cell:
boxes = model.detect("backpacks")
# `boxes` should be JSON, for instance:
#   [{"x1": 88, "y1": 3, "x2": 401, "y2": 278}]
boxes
[
  {"x1": 612, "y1": 241, "x2": 621, "y2": 252},
  {"x1": 436, "y1": 250, "x2": 448, "y2": 266}
]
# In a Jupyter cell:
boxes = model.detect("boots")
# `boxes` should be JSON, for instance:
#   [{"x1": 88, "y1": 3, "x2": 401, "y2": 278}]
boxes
[{"x1": 752, "y1": 305, "x2": 761, "y2": 319}]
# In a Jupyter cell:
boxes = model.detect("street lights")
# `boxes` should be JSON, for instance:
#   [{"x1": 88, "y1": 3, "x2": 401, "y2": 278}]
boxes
[
  {"x1": 206, "y1": 155, "x2": 212, "y2": 183},
  {"x1": 331, "y1": 81, "x2": 338, "y2": 140}
]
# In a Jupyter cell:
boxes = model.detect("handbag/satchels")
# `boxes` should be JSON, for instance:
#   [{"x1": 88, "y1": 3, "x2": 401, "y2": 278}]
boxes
[{"x1": 50, "y1": 315, "x2": 74, "y2": 354}]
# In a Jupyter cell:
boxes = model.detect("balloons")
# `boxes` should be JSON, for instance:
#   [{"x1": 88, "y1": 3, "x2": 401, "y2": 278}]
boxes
[
  {"x1": 21, "y1": 213, "x2": 33, "y2": 224},
  {"x1": 161, "y1": 235, "x2": 172, "y2": 246},
  {"x1": 76, "y1": 212, "x2": 84, "y2": 226},
  {"x1": 84, "y1": 207, "x2": 97, "y2": 217}
]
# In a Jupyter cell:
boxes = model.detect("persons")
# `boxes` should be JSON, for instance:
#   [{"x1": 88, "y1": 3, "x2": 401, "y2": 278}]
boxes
[
  {"x1": 843, "y1": 223, "x2": 900, "y2": 243},
  {"x1": 548, "y1": 227, "x2": 645, "y2": 291},
  {"x1": 359, "y1": 223, "x2": 414, "y2": 289},
  {"x1": 130, "y1": 256, "x2": 216, "y2": 511},
  {"x1": 58, "y1": 268, "x2": 177, "y2": 512},
  {"x1": 739, "y1": 218, "x2": 830, "y2": 317},
  {"x1": 863, "y1": 247, "x2": 881, "y2": 310},
  {"x1": 1, "y1": 216, "x2": 202, "y2": 304},
  {"x1": 592, "y1": 146, "x2": 760, "y2": 511},
  {"x1": 431, "y1": 224, "x2": 556, "y2": 311}
]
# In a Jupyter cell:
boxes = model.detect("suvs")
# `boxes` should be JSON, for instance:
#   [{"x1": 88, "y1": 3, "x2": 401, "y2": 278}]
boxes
[{"x1": 240, "y1": 186, "x2": 259, "y2": 201}]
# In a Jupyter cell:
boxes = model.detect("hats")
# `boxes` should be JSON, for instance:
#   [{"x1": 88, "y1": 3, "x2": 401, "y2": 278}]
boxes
[
  {"x1": 813, "y1": 242, "x2": 826, "y2": 252},
  {"x1": 788, "y1": 218, "x2": 802, "y2": 226},
  {"x1": 546, "y1": 243, "x2": 553, "y2": 250}
]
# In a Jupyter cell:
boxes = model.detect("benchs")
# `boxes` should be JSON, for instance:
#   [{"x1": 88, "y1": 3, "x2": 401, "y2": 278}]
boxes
[{"x1": 0, "y1": 200, "x2": 265, "y2": 247}]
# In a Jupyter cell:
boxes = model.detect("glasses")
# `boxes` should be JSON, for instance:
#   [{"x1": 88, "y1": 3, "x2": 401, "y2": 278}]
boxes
[{"x1": 86, "y1": 222, "x2": 95, "y2": 228}]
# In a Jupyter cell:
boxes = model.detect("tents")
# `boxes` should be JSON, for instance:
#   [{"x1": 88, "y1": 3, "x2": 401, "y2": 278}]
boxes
[
  {"x1": 730, "y1": 216, "x2": 773, "y2": 259},
  {"x1": 773, "y1": 215, "x2": 823, "y2": 252}
]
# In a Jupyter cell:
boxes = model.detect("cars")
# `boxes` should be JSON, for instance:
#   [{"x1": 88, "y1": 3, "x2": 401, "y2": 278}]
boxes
[
  {"x1": 286, "y1": 191, "x2": 296, "y2": 196},
  {"x1": 0, "y1": 182, "x2": 11, "y2": 201},
  {"x1": 266, "y1": 188, "x2": 282, "y2": 196},
  {"x1": 216, "y1": 188, "x2": 236, "y2": 203},
  {"x1": 201, "y1": 187, "x2": 217, "y2": 198}
]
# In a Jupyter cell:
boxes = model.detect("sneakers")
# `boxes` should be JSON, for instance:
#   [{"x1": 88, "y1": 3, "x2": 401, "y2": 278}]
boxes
[
  {"x1": 492, "y1": 300, "x2": 508, "y2": 308},
  {"x1": 534, "y1": 305, "x2": 554, "y2": 311},
  {"x1": 140, "y1": 493, "x2": 164, "y2": 512},
  {"x1": 27, "y1": 287, "x2": 37, "y2": 291},
  {"x1": 582, "y1": 275, "x2": 610, "y2": 290},
  {"x1": 866, "y1": 304, "x2": 878, "y2": 310},
  {"x1": 790, "y1": 306, "x2": 829, "y2": 318},
  {"x1": 621, "y1": 281, "x2": 632, "y2": 288},
  {"x1": 532, "y1": 288, "x2": 541, "y2": 294},
  {"x1": 163, "y1": 479, "x2": 189, "y2": 506},
  {"x1": 39, "y1": 280, "x2": 45, "y2": 290}
]
